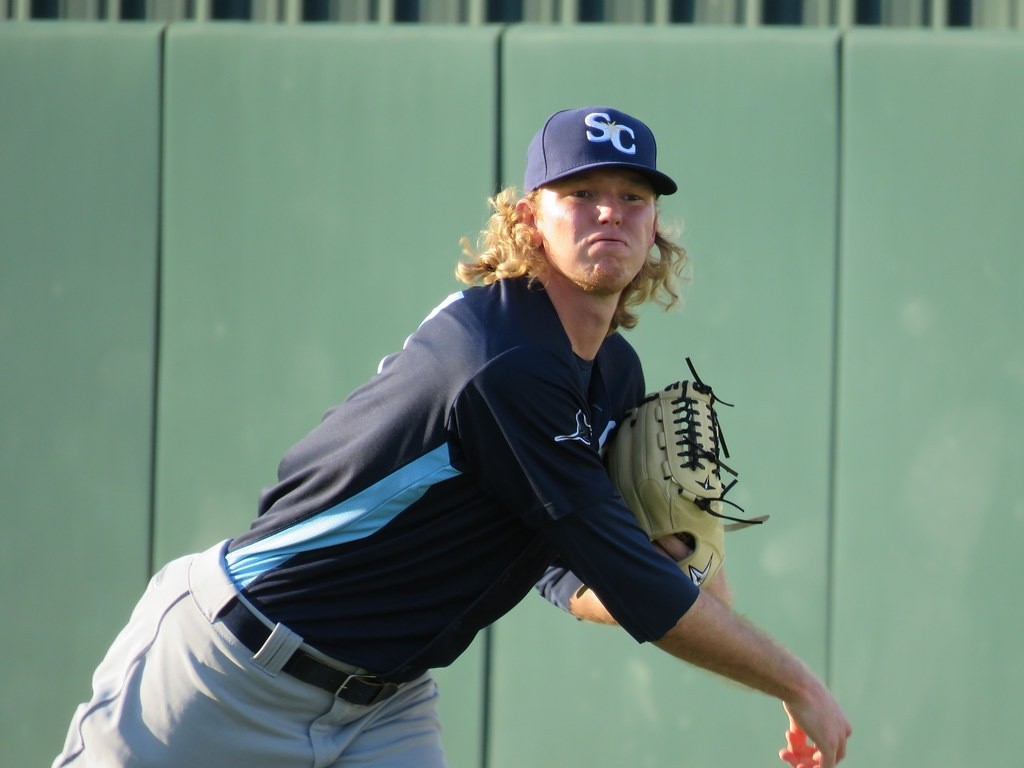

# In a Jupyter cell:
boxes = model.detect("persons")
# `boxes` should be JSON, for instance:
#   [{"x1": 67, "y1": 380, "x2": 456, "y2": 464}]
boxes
[{"x1": 46, "y1": 107, "x2": 852, "y2": 768}]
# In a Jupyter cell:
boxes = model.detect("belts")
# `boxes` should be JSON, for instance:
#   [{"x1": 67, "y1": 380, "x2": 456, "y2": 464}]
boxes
[{"x1": 217, "y1": 598, "x2": 399, "y2": 706}]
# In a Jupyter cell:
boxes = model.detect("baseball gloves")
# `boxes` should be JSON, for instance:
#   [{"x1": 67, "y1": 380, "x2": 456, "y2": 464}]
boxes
[{"x1": 605, "y1": 378, "x2": 728, "y2": 595}]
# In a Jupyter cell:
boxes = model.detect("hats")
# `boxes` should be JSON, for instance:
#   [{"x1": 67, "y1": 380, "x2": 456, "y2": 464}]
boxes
[{"x1": 524, "y1": 106, "x2": 677, "y2": 195}]
